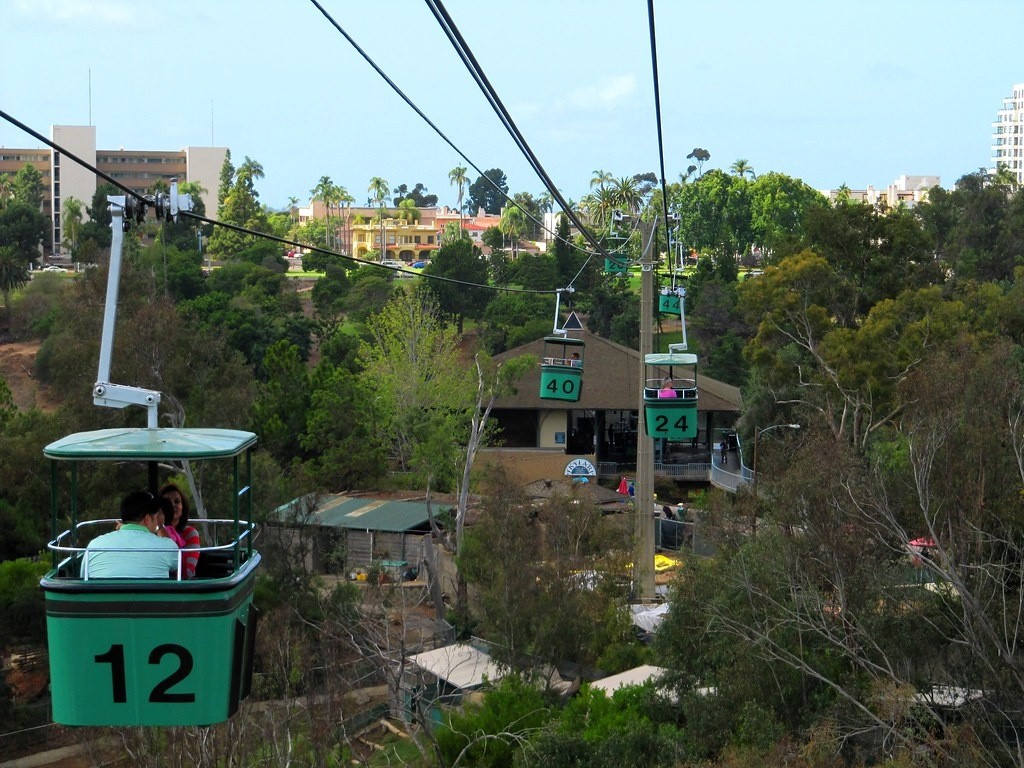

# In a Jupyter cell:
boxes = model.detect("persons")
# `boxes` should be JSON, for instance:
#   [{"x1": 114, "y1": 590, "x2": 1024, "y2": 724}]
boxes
[
  {"x1": 379, "y1": 568, "x2": 392, "y2": 582},
  {"x1": 720, "y1": 439, "x2": 728, "y2": 463},
  {"x1": 350, "y1": 569, "x2": 366, "y2": 581},
  {"x1": 405, "y1": 566, "x2": 417, "y2": 581},
  {"x1": 608, "y1": 424, "x2": 615, "y2": 446},
  {"x1": 660, "y1": 376, "x2": 676, "y2": 398},
  {"x1": 80, "y1": 484, "x2": 200, "y2": 579},
  {"x1": 566, "y1": 353, "x2": 579, "y2": 366},
  {"x1": 921, "y1": 547, "x2": 929, "y2": 568}
]
